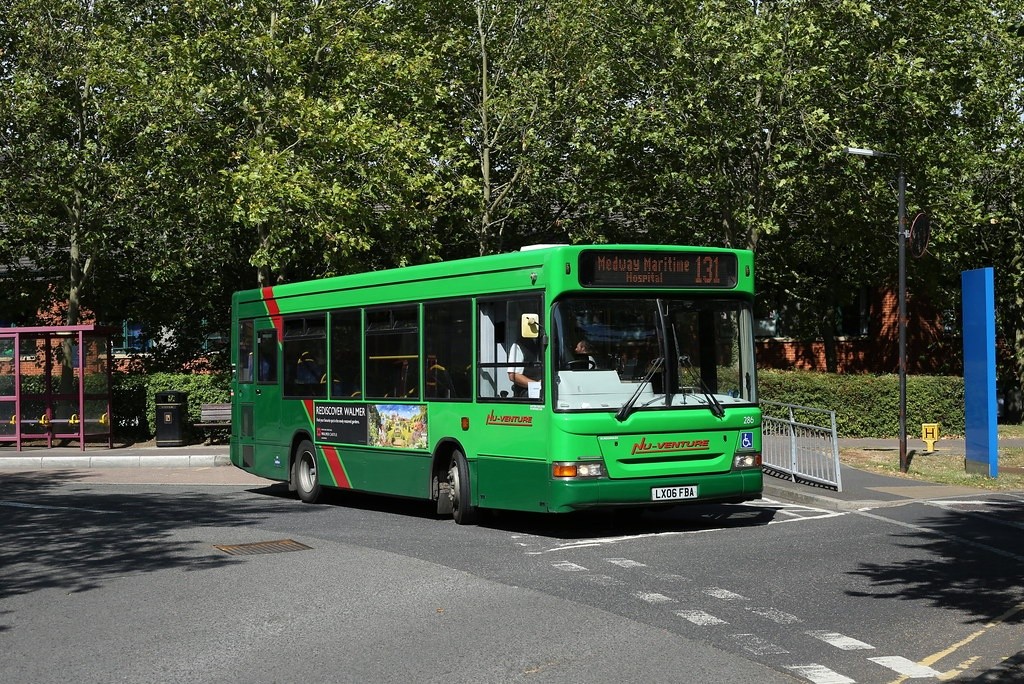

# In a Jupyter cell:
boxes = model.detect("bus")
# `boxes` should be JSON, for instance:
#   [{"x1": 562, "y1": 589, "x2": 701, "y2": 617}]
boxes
[{"x1": 229, "y1": 244, "x2": 762, "y2": 525}]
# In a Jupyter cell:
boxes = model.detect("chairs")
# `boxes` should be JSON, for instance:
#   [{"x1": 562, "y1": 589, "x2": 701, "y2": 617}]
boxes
[{"x1": 247, "y1": 347, "x2": 458, "y2": 399}]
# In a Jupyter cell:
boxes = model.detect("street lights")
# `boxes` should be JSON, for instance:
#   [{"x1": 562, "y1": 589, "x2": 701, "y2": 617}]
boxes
[{"x1": 842, "y1": 147, "x2": 906, "y2": 473}]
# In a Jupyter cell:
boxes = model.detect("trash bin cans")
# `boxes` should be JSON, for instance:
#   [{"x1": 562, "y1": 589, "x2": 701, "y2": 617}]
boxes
[{"x1": 154, "y1": 391, "x2": 190, "y2": 447}]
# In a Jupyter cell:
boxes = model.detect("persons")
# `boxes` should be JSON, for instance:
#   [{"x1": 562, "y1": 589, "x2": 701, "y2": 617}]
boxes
[
  {"x1": 379, "y1": 424, "x2": 385, "y2": 434},
  {"x1": 621, "y1": 347, "x2": 641, "y2": 369},
  {"x1": 575, "y1": 340, "x2": 597, "y2": 370},
  {"x1": 507, "y1": 316, "x2": 557, "y2": 397}
]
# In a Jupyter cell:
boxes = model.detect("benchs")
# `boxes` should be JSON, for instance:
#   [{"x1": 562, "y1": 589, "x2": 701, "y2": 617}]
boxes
[{"x1": 194, "y1": 402, "x2": 232, "y2": 446}]
